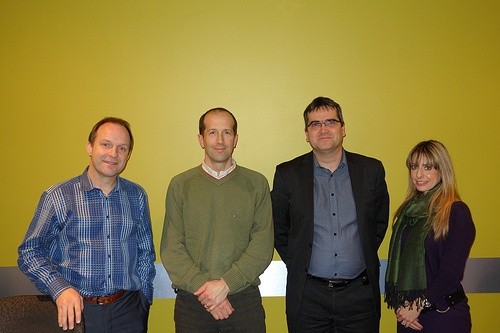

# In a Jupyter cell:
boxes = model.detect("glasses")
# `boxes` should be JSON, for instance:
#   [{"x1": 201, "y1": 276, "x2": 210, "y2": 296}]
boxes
[{"x1": 305, "y1": 118, "x2": 343, "y2": 128}]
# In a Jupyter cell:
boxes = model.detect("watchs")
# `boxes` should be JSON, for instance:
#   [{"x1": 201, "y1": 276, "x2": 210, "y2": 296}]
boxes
[{"x1": 422, "y1": 294, "x2": 431, "y2": 307}]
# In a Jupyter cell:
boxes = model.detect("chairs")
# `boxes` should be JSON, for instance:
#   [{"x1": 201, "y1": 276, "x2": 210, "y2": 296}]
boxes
[{"x1": 0, "y1": 295, "x2": 84, "y2": 333}]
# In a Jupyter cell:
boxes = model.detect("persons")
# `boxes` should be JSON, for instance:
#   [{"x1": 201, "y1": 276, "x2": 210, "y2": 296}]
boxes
[
  {"x1": 269, "y1": 96, "x2": 390, "y2": 333},
  {"x1": 385, "y1": 139, "x2": 476, "y2": 333},
  {"x1": 16, "y1": 116, "x2": 156, "y2": 333},
  {"x1": 160, "y1": 108, "x2": 275, "y2": 333}
]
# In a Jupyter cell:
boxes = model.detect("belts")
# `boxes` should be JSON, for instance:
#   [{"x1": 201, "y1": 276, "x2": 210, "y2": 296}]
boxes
[
  {"x1": 307, "y1": 273, "x2": 370, "y2": 287},
  {"x1": 84, "y1": 289, "x2": 125, "y2": 305}
]
